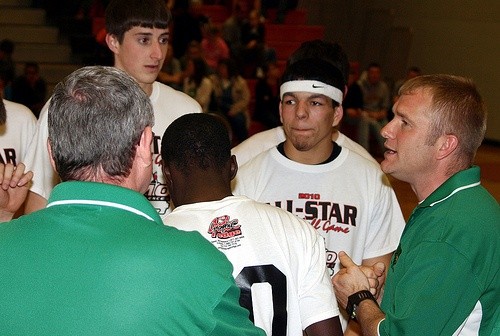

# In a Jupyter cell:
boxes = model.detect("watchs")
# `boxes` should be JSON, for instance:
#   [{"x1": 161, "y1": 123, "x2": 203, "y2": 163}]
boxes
[{"x1": 346, "y1": 290, "x2": 376, "y2": 319}]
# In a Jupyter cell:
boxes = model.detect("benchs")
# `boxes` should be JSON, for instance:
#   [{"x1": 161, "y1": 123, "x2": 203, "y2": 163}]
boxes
[{"x1": 0, "y1": 0, "x2": 359, "y2": 135}]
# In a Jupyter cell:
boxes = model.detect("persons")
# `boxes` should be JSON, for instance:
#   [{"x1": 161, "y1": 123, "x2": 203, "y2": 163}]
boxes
[
  {"x1": 21, "y1": 1, "x2": 202, "y2": 222},
  {"x1": 332, "y1": 74, "x2": 500, "y2": 336},
  {"x1": 229, "y1": 59, "x2": 406, "y2": 336},
  {"x1": 65, "y1": 4, "x2": 114, "y2": 68},
  {"x1": 160, "y1": 113, "x2": 344, "y2": 336},
  {"x1": 0, "y1": 67, "x2": 268, "y2": 336},
  {"x1": 0, "y1": 39, "x2": 47, "y2": 172},
  {"x1": 339, "y1": 60, "x2": 422, "y2": 160},
  {"x1": 156, "y1": 0, "x2": 284, "y2": 147}
]
